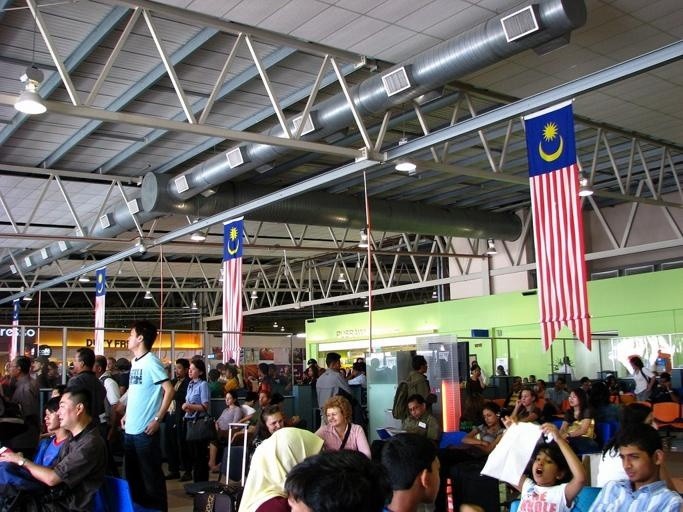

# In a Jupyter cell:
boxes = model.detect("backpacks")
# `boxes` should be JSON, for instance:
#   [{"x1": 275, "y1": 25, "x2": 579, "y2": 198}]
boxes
[{"x1": 391, "y1": 379, "x2": 412, "y2": 420}]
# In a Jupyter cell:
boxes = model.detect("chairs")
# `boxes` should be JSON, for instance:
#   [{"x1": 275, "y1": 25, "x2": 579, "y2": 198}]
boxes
[
  {"x1": 94, "y1": 396, "x2": 148, "y2": 512},
  {"x1": 186, "y1": 383, "x2": 682, "y2": 512}
]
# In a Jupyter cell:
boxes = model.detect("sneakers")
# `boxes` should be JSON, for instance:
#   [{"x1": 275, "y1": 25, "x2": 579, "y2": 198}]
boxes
[{"x1": 163, "y1": 470, "x2": 207, "y2": 494}]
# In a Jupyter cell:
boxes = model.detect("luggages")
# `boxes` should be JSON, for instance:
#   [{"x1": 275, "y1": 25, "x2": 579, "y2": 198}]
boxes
[{"x1": 192, "y1": 483, "x2": 244, "y2": 511}]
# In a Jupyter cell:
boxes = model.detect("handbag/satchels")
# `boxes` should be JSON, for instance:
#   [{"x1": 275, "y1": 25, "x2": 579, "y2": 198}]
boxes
[
  {"x1": 187, "y1": 406, "x2": 218, "y2": 442},
  {"x1": 565, "y1": 418, "x2": 596, "y2": 440}
]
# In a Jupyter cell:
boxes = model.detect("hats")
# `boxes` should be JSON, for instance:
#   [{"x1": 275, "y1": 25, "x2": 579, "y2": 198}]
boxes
[{"x1": 32, "y1": 355, "x2": 50, "y2": 364}]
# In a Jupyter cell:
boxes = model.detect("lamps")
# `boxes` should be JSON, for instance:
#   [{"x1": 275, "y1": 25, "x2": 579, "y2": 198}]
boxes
[
  {"x1": 363, "y1": 300, "x2": 370, "y2": 308},
  {"x1": 189, "y1": 217, "x2": 206, "y2": 242},
  {"x1": 12, "y1": 3, "x2": 48, "y2": 115},
  {"x1": 143, "y1": 289, "x2": 152, "y2": 300},
  {"x1": 218, "y1": 268, "x2": 226, "y2": 282},
  {"x1": 431, "y1": 288, "x2": 438, "y2": 300},
  {"x1": 190, "y1": 299, "x2": 199, "y2": 310},
  {"x1": 281, "y1": 326, "x2": 285, "y2": 332},
  {"x1": 79, "y1": 266, "x2": 90, "y2": 283},
  {"x1": 577, "y1": 168, "x2": 595, "y2": 197},
  {"x1": 337, "y1": 269, "x2": 347, "y2": 283},
  {"x1": 356, "y1": 227, "x2": 371, "y2": 249},
  {"x1": 484, "y1": 237, "x2": 498, "y2": 256},
  {"x1": 250, "y1": 289, "x2": 258, "y2": 299},
  {"x1": 392, "y1": 100, "x2": 418, "y2": 173},
  {"x1": 23, "y1": 289, "x2": 32, "y2": 301},
  {"x1": 273, "y1": 322, "x2": 278, "y2": 328},
  {"x1": 24, "y1": 257, "x2": 32, "y2": 268}
]
